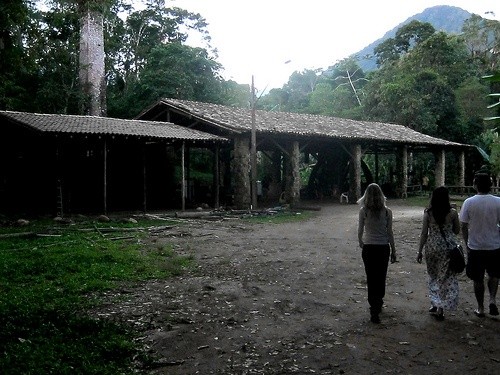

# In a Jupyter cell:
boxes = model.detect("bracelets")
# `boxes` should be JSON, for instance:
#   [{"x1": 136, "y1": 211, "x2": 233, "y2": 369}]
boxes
[{"x1": 418, "y1": 252, "x2": 421, "y2": 253}]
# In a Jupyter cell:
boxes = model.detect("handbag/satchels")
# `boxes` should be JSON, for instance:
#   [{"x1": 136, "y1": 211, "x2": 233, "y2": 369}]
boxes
[{"x1": 449, "y1": 244, "x2": 465, "y2": 273}]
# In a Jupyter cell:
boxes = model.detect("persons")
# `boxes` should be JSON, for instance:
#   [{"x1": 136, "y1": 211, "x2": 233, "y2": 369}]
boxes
[
  {"x1": 357, "y1": 183, "x2": 397, "y2": 323},
  {"x1": 417, "y1": 186, "x2": 461, "y2": 319},
  {"x1": 422, "y1": 174, "x2": 429, "y2": 196},
  {"x1": 459, "y1": 174, "x2": 500, "y2": 316}
]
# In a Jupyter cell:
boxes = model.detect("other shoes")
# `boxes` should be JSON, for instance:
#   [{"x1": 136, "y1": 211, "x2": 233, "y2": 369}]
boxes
[
  {"x1": 473, "y1": 309, "x2": 485, "y2": 318},
  {"x1": 488, "y1": 303, "x2": 499, "y2": 316},
  {"x1": 370, "y1": 316, "x2": 381, "y2": 324},
  {"x1": 428, "y1": 306, "x2": 443, "y2": 315}
]
